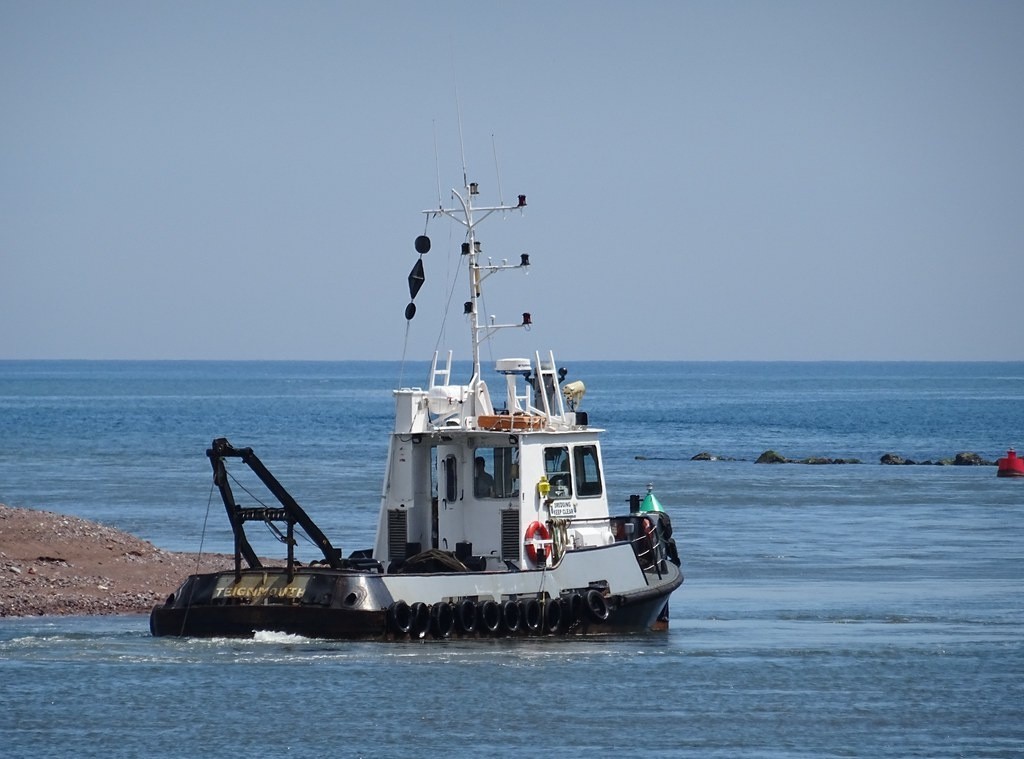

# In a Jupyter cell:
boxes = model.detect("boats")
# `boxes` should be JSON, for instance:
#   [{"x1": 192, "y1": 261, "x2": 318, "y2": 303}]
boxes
[{"x1": 149, "y1": 93, "x2": 688, "y2": 642}]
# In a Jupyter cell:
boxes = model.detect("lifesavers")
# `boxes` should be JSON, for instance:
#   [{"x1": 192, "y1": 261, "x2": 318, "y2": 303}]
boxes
[{"x1": 526, "y1": 520, "x2": 553, "y2": 560}]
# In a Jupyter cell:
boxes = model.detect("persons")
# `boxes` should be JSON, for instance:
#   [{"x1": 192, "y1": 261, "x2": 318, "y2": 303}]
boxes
[
  {"x1": 475, "y1": 456, "x2": 494, "y2": 498},
  {"x1": 548, "y1": 458, "x2": 569, "y2": 487}
]
